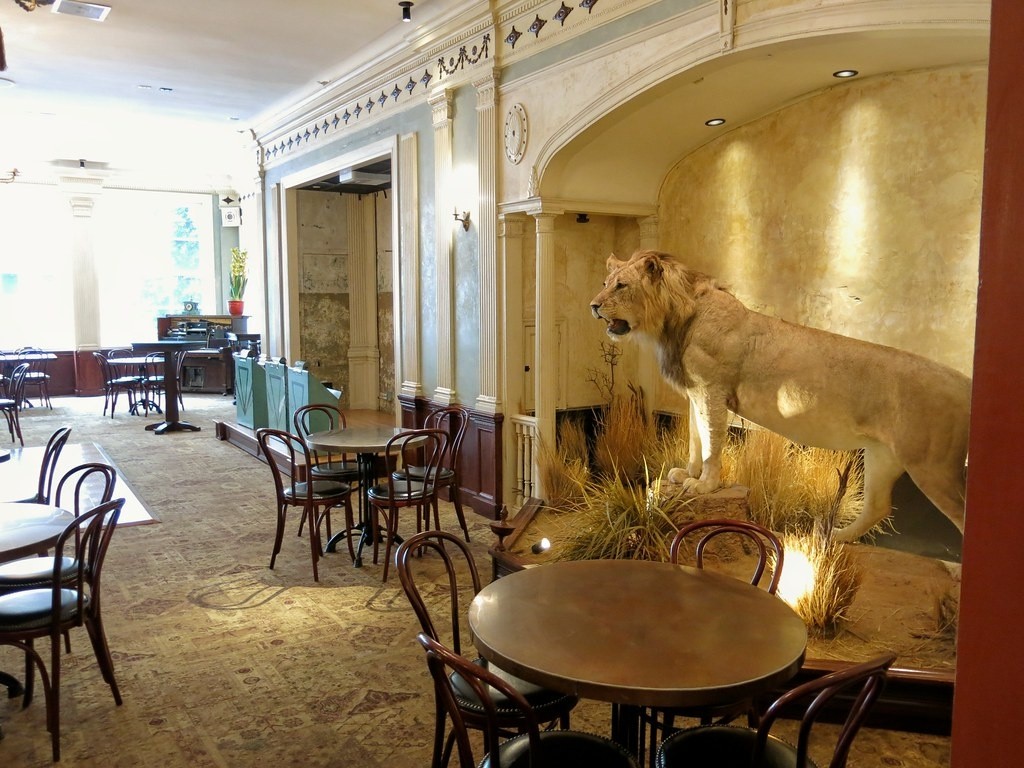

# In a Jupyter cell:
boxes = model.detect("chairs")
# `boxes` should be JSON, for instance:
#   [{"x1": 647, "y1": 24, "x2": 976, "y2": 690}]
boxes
[
  {"x1": 656, "y1": 652, "x2": 898, "y2": 768},
  {"x1": 366, "y1": 427, "x2": 450, "y2": 583},
  {"x1": 641, "y1": 518, "x2": 784, "y2": 768},
  {"x1": 394, "y1": 529, "x2": 582, "y2": 768},
  {"x1": 293, "y1": 403, "x2": 367, "y2": 544},
  {"x1": 392, "y1": 407, "x2": 472, "y2": 543},
  {"x1": 254, "y1": 427, "x2": 356, "y2": 582},
  {"x1": 415, "y1": 631, "x2": 641, "y2": 768},
  {"x1": 0, "y1": 344, "x2": 189, "y2": 763}
]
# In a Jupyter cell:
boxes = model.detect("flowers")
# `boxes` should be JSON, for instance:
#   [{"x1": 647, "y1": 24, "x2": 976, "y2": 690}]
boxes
[{"x1": 226, "y1": 246, "x2": 249, "y2": 300}]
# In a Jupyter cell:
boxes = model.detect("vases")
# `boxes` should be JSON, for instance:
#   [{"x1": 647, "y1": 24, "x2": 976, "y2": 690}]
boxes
[{"x1": 228, "y1": 300, "x2": 245, "y2": 316}]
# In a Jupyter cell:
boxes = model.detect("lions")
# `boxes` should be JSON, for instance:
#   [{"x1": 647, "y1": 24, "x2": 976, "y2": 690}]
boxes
[{"x1": 589, "y1": 249, "x2": 973, "y2": 543}]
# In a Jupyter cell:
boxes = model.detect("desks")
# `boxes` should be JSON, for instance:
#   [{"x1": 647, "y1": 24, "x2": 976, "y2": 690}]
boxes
[
  {"x1": 304, "y1": 427, "x2": 431, "y2": 568},
  {"x1": 130, "y1": 341, "x2": 208, "y2": 434},
  {"x1": 466, "y1": 558, "x2": 809, "y2": 758},
  {"x1": 107, "y1": 357, "x2": 178, "y2": 416},
  {"x1": 0, "y1": 441, "x2": 164, "y2": 534},
  {"x1": 0, "y1": 353, "x2": 58, "y2": 409}
]
